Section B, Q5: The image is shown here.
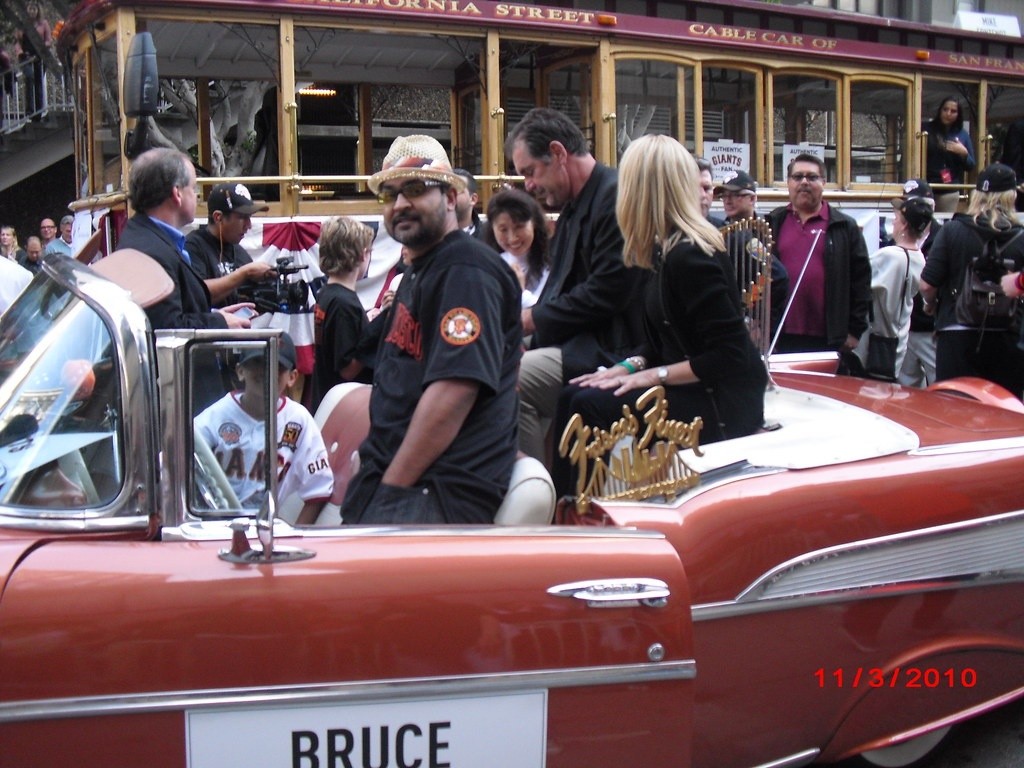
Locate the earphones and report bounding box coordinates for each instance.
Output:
[216,214,221,223]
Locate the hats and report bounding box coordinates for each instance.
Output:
[239,328,296,372]
[977,161,1018,192]
[207,179,269,215]
[713,170,757,191]
[367,134,467,193]
[899,178,933,198]
[891,196,933,231]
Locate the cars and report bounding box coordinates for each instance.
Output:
[0,252,1023,768]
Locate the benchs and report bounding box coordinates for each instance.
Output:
[276,381,556,526]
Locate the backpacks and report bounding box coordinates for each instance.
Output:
[945,216,1024,352]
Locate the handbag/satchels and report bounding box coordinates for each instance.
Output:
[866,329,899,381]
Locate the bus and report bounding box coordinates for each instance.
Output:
[50,1,1024,264]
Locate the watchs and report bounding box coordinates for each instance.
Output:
[655,364,669,385]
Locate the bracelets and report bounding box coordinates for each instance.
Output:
[615,360,635,373]
[625,357,645,370]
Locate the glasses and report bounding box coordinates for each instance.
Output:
[718,193,750,202]
[377,179,448,204]
[789,173,823,182]
[39,226,57,230]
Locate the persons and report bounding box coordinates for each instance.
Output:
[690,156,789,320]
[762,153,872,375]
[341,133,521,526]
[508,108,663,464]
[184,182,276,308]
[13,2,53,122]
[0,226,26,264]
[917,94,976,214]
[46,215,74,260]
[192,327,335,525]
[859,195,932,380]
[18,235,47,274]
[544,132,768,508]
[1000,268,1024,298]
[119,149,257,329]
[712,169,766,231]
[38,218,58,252]
[884,179,945,388]
[921,163,1024,400]
[313,166,559,386]
